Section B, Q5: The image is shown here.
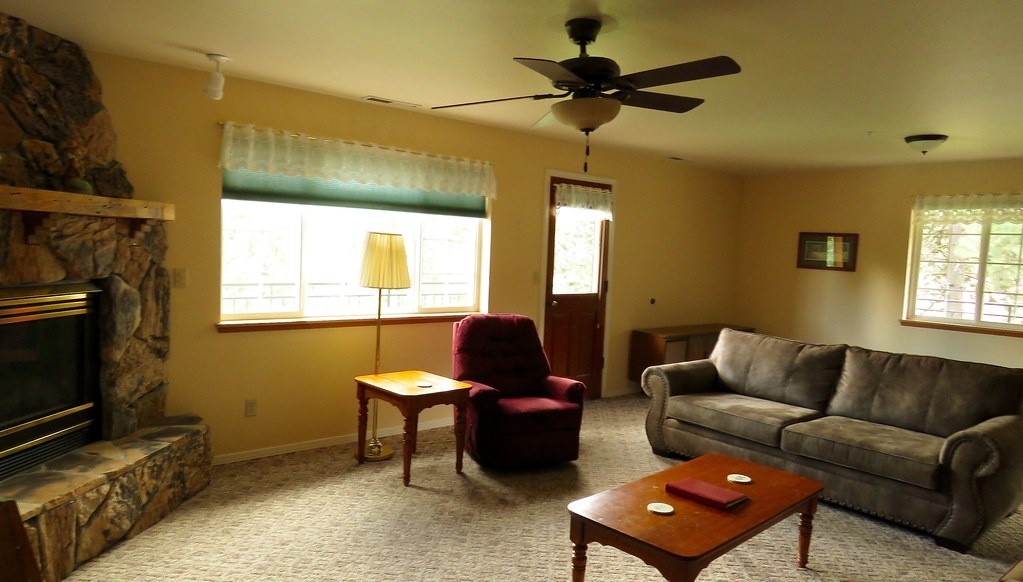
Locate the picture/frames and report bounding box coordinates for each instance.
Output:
[794,231,860,271]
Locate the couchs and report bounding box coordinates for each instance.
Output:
[451,310,586,477]
[640,309,1023,552]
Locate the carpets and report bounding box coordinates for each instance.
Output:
[61,380,1022,582]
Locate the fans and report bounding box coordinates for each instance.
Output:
[431,0,744,178]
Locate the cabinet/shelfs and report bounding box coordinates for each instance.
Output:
[627,321,755,389]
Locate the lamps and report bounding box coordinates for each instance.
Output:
[352,232,413,462]
[202,52,231,101]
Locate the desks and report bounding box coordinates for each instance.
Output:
[354,368,473,488]
[565,452,827,581]
